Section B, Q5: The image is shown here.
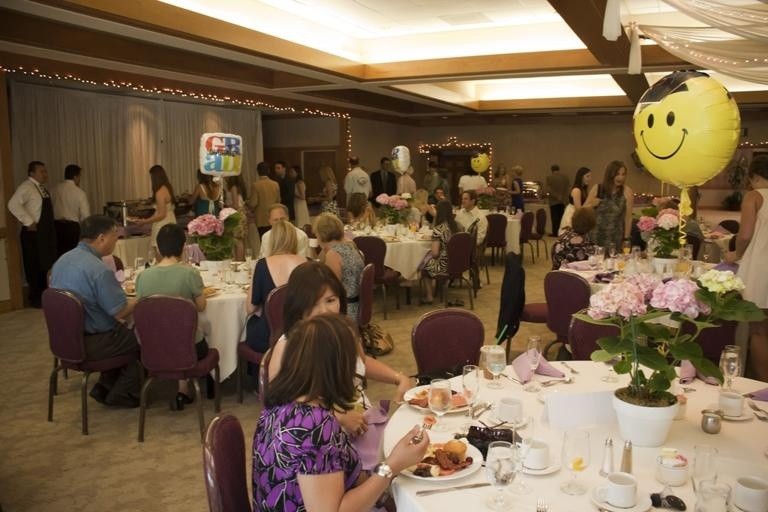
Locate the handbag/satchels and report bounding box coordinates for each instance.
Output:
[359,320,394,356]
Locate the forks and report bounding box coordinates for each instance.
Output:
[408,423,432,445]
[747,400,768,415]
[534,496,548,512]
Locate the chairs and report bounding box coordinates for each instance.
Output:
[544,270,590,360]
[411,307,485,387]
[202,410,253,512]
[299,209,549,320]
[568,316,621,360]
[42,243,375,441]
[495,252,549,365]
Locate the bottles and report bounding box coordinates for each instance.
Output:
[619,439,634,474]
[658,448,690,487]
[695,479,732,511]
[599,436,613,476]
[673,393,688,421]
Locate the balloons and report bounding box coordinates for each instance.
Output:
[632,70,741,246]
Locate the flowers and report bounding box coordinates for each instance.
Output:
[376,192,416,225]
[631,207,685,257]
[476,186,496,209]
[187,209,247,261]
[571,269,767,399]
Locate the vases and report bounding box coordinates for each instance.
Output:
[199,259,233,283]
[652,257,681,277]
[612,386,680,448]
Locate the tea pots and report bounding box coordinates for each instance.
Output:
[692,443,718,491]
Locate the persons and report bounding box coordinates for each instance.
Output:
[544,159,705,271]
[726,155,768,382]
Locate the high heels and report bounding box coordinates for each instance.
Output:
[175,393,193,410]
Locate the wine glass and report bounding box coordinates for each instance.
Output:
[485,440,518,510]
[487,345,507,389]
[120,248,254,294]
[603,351,622,384]
[558,429,593,495]
[588,241,705,279]
[719,342,743,392]
[460,364,480,430]
[429,379,452,433]
[523,336,543,392]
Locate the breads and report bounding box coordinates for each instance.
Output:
[443,440,467,462]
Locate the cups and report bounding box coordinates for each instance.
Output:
[498,398,522,424]
[479,346,500,381]
[719,390,744,418]
[701,407,723,434]
[733,477,768,512]
[598,471,638,509]
[521,437,549,469]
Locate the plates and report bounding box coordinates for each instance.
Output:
[400,435,484,482]
[590,484,652,511]
[518,450,560,474]
[402,384,477,415]
[720,408,754,422]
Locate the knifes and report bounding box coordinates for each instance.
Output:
[416,483,493,500]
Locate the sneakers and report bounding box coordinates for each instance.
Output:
[89,384,141,408]
[420,297,432,305]
